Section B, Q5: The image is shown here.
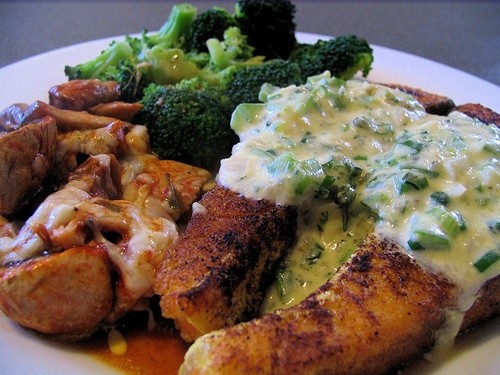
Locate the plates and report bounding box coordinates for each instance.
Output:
[0,31,500,375]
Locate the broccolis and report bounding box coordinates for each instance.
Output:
[62,0,374,171]
